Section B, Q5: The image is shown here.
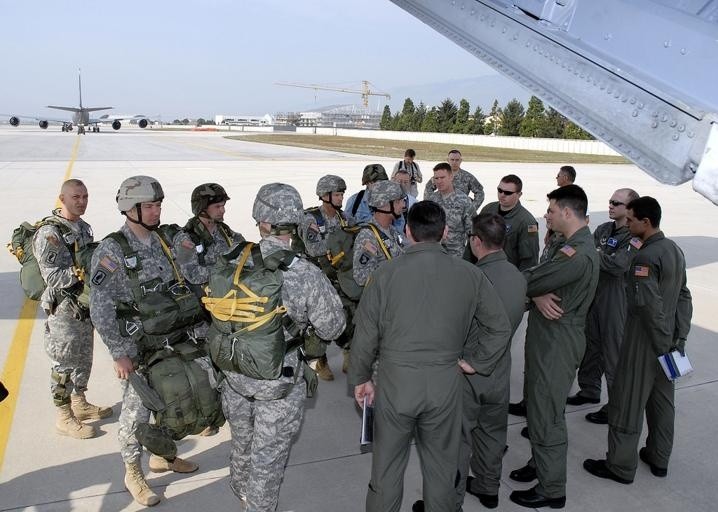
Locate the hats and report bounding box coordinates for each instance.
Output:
[315,175,348,198]
[367,181,407,207]
[191,182,232,212]
[361,163,389,185]
[250,182,308,224]
[117,175,165,212]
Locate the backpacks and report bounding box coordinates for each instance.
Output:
[327,221,391,300]
[9,216,75,299]
[78,230,139,311]
[205,243,307,381]
[161,221,199,244]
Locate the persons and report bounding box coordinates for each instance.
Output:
[349,200,526,512]
[90,175,347,512]
[31,179,113,440]
[295,149,540,454]
[508,164,692,510]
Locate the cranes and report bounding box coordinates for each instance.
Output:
[270,78,394,114]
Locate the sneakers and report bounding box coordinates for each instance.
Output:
[342,350,351,372]
[308,355,336,383]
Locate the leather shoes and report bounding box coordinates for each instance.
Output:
[566,392,602,407]
[640,446,667,477]
[510,485,565,510]
[509,466,536,482]
[583,456,635,486]
[466,473,500,509]
[508,399,528,417]
[585,409,611,426]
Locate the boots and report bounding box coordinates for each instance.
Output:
[54,404,97,440]
[123,460,161,509]
[147,452,201,475]
[71,393,113,420]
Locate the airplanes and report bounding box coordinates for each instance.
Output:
[0,68,164,133]
[381,1,718,214]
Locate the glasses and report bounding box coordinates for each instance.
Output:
[608,198,627,207]
[498,187,517,196]
